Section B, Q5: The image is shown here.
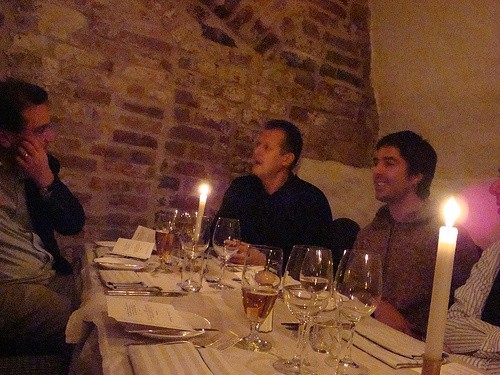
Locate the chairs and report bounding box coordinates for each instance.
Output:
[329,218,361,279]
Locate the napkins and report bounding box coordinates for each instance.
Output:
[97,269,162,293]
[338,320,450,370]
[127,343,237,375]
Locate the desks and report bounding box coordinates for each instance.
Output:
[65,244,491,375]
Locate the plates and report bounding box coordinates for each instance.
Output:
[220,272,281,295]
[277,285,344,312]
[121,310,212,339]
[93,258,149,271]
[94,241,117,247]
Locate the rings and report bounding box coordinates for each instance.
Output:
[23,152,28,157]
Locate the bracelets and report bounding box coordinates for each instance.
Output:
[40,180,57,195]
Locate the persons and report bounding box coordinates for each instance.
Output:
[0,76,84,342]
[444,169,500,373]
[349,129,482,339]
[210,119,333,275]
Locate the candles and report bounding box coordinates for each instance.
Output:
[194,182,211,241]
[420,195,461,375]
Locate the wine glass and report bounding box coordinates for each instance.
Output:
[236,245,283,352]
[209,217,241,290]
[272,244,383,375]
[152,207,177,274]
[174,211,212,288]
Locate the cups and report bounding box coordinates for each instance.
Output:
[206,244,222,282]
[182,251,205,292]
[225,241,250,272]
[310,316,343,354]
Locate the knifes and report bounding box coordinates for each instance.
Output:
[125,329,220,333]
[107,290,189,296]
[281,322,355,326]
[286,326,354,330]
